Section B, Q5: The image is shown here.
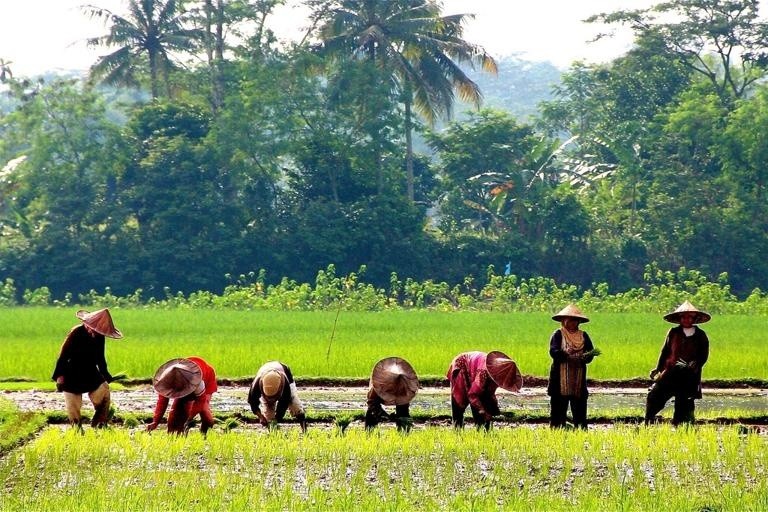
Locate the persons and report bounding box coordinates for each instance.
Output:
[547,304,594,432]
[52,307,124,430]
[645,300,711,427]
[447,350,523,431]
[248,361,306,433]
[146,356,217,434]
[365,356,420,433]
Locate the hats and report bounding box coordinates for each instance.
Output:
[76,308,123,339]
[372,357,419,406]
[263,372,281,396]
[153,358,202,400]
[552,304,589,323]
[485,351,522,392]
[664,300,711,324]
